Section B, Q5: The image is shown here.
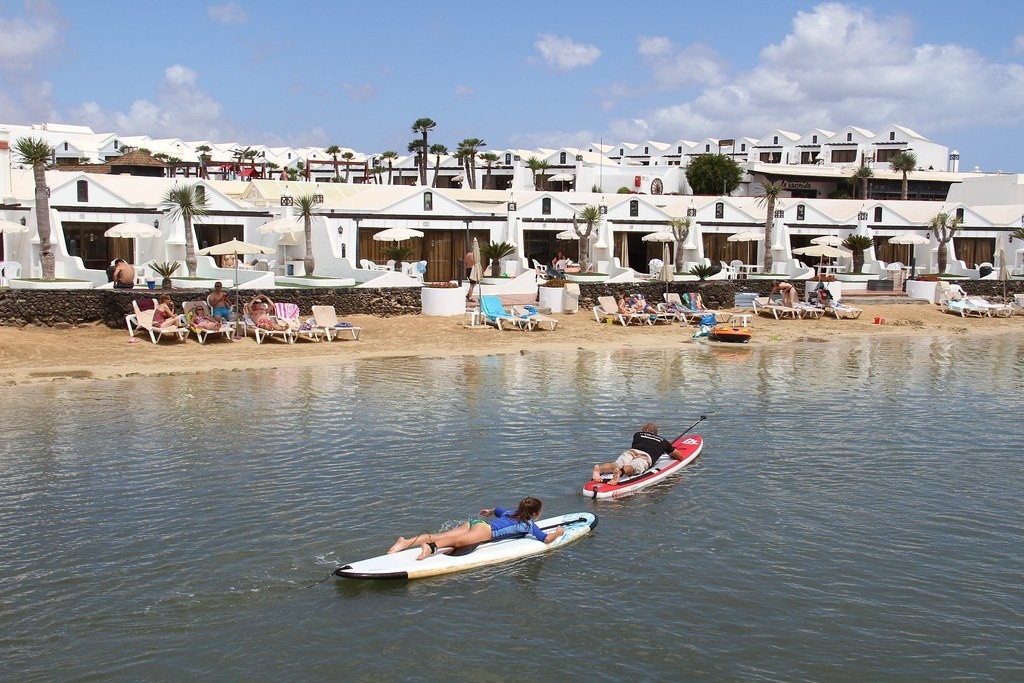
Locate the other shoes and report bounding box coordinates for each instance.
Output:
[466,294,476,302]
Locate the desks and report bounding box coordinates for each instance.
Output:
[733,315,754,328]
[464,312,486,328]
[223,320,247,337]
[813,265,845,277]
[806,303,822,317]
[740,265,763,273]
[902,266,925,279]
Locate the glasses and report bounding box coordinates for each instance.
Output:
[197,309,204,311]
[215,285,220,287]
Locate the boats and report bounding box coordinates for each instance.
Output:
[579,430,707,502]
[336,510,603,582]
[708,325,752,342]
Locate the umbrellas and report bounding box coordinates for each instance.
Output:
[791,234,852,309]
[641,230,683,265]
[547,170,575,191]
[0,218,29,234]
[195,237,276,338]
[888,233,930,269]
[659,243,674,313]
[372,227,424,250]
[727,229,765,273]
[556,227,597,263]
[257,218,314,276]
[993,233,1012,307]
[463,236,494,330]
[104,221,161,265]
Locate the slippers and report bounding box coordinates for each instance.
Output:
[128,339,139,343]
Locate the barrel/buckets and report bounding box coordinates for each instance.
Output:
[606,319,612,326]
[874,317,880,324]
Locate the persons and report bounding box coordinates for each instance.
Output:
[552,252,566,270]
[386,495,564,561]
[113,254,288,340]
[768,256,833,318]
[464,245,477,302]
[592,423,683,486]
[618,294,658,326]
[281,167,288,181]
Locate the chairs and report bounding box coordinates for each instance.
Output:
[478,296,558,332]
[360,258,427,282]
[125,298,361,345]
[111,258,155,285]
[0,262,22,287]
[944,291,1024,318]
[800,262,809,268]
[532,259,547,272]
[593,293,733,326]
[556,258,570,270]
[720,259,744,280]
[648,259,662,275]
[753,297,863,320]
